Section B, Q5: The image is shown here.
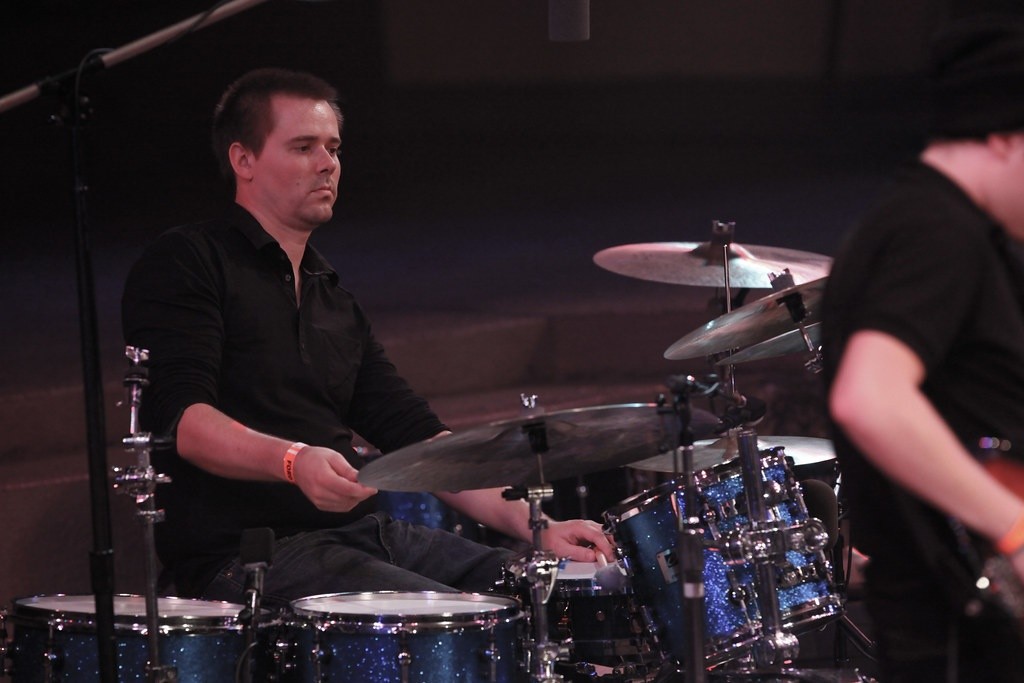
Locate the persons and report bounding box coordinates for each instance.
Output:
[816,79,1024,683]
[121,68,621,660]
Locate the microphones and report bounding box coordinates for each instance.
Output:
[241,527,275,606]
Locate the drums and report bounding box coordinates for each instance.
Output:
[11,452,842,682]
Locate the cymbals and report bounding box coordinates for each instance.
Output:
[357,244,835,496]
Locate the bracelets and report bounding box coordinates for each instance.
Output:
[997,517,1024,556]
[284,442,307,482]
